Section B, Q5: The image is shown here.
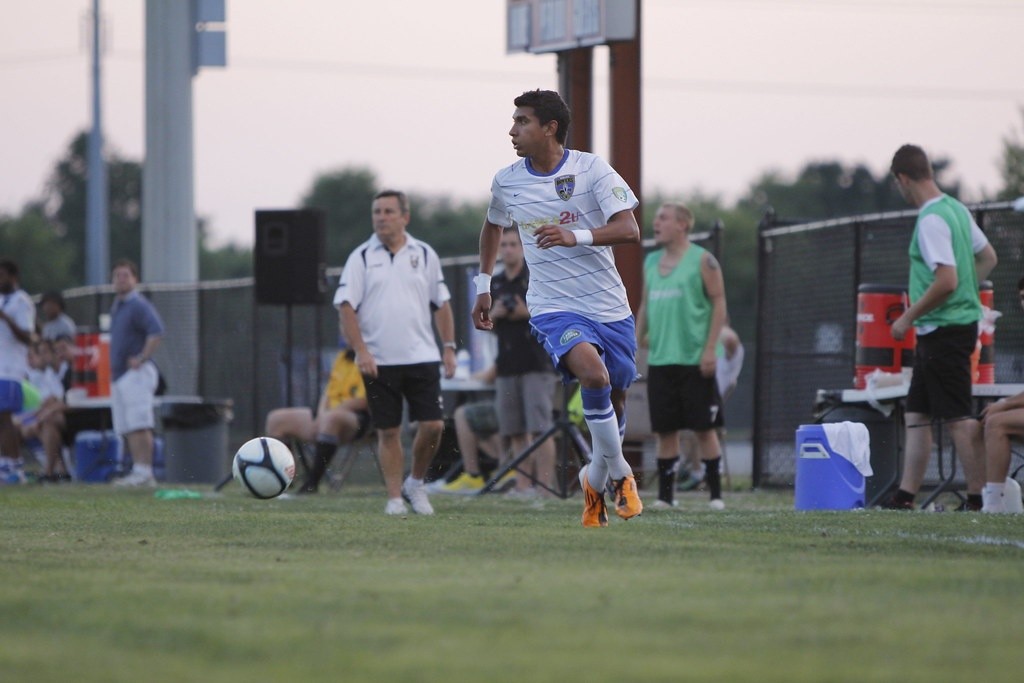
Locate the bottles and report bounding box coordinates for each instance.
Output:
[455,347,471,377]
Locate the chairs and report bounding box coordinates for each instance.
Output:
[287,426,387,492]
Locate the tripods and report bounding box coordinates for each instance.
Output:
[477,381,617,502]
[213,306,312,493]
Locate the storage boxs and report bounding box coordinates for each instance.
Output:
[69,432,163,480]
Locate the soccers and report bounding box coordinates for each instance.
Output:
[231,435,295,500]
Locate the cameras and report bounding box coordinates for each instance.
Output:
[499,294,518,312]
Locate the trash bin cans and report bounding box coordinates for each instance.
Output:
[795,423,866,512]
[153,395,233,484]
[63,397,123,484]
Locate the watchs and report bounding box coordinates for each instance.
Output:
[443,342,456,349]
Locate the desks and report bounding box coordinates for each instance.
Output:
[439,376,497,481]
[815,383,1024,510]
[67,394,234,409]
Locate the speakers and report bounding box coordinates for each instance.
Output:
[253,209,327,306]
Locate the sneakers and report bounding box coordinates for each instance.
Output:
[579,464,610,528]
[606,472,642,521]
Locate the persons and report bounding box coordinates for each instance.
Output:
[427,90,644,529]
[635,201,745,512]
[107,259,163,488]
[0,261,29,486]
[263,185,458,517]
[979,281,1024,513]
[876,145,998,510]
[8,290,113,485]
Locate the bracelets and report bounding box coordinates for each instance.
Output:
[571,229,593,246]
[472,273,492,295]
[137,353,145,362]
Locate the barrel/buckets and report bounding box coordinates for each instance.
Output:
[978,281,995,384]
[75,432,123,482]
[855,284,914,388]
[795,424,865,511]
[96,334,111,396]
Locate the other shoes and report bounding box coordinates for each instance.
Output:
[57,473,72,484]
[401,478,432,515]
[710,499,724,511]
[954,500,982,512]
[0,465,10,480]
[494,470,518,489]
[38,475,57,484]
[385,499,406,515]
[6,472,27,484]
[444,472,485,495]
[425,479,445,492]
[113,472,156,486]
[650,499,677,511]
[882,497,914,511]
